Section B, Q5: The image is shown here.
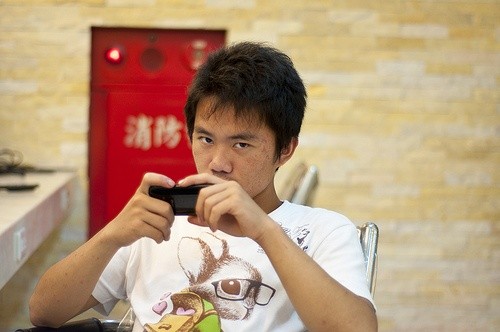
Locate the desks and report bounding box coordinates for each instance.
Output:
[0,169,77,291]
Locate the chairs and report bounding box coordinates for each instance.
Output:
[281,161,319,207]
[15,222,379,332]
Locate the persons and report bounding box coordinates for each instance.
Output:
[28,41,379,332]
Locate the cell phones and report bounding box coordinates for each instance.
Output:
[149,184,209,216]
